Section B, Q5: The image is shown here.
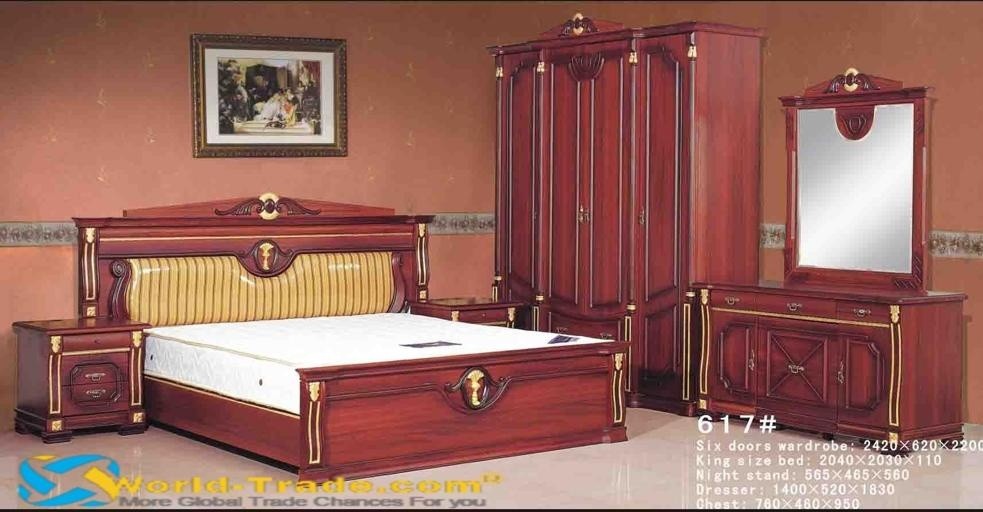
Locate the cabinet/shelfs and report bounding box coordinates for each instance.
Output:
[485,11,969,459]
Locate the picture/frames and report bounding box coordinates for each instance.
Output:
[190,32,349,158]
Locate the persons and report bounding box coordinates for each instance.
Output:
[251,79,314,128]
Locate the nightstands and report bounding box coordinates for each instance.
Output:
[11,318,152,444]
[407,297,525,328]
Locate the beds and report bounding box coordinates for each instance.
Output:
[70,192,633,488]
